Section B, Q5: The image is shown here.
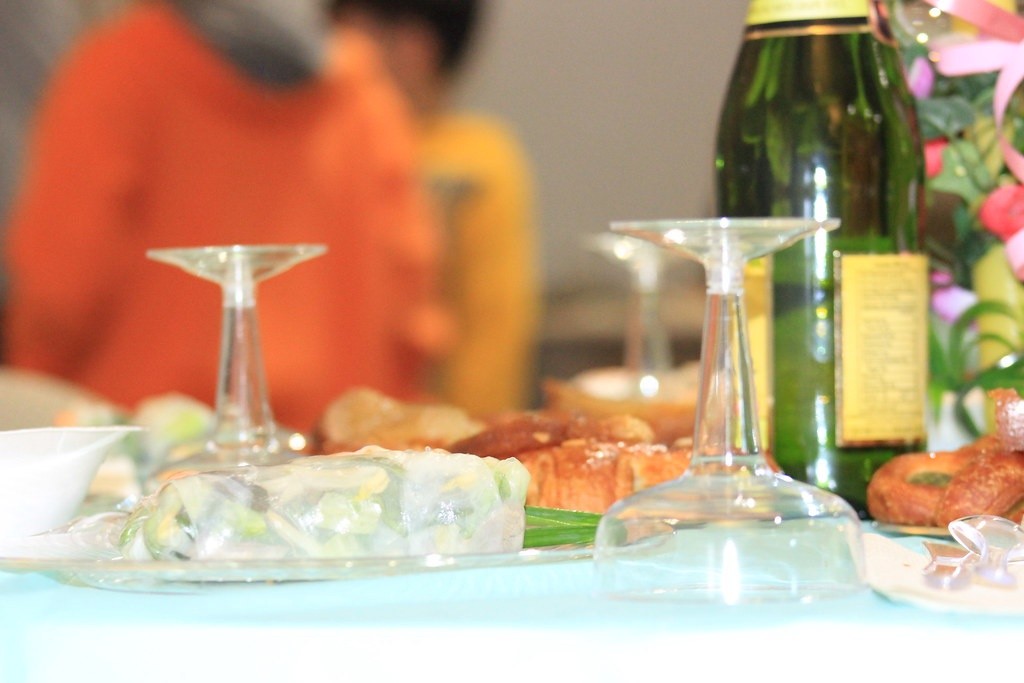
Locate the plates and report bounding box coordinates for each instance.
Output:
[0,516,675,584]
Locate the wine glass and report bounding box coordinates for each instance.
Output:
[141,245,312,497]
[592,216,866,599]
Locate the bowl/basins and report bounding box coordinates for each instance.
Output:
[0,425,129,537]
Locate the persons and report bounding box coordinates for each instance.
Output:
[0,0,436,450]
[321,1,539,423]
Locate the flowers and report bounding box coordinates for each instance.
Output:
[874,0,1023,440]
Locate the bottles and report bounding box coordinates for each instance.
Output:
[705,0,931,522]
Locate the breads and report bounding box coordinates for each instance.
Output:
[866,389,1023,533]
[318,387,785,518]
